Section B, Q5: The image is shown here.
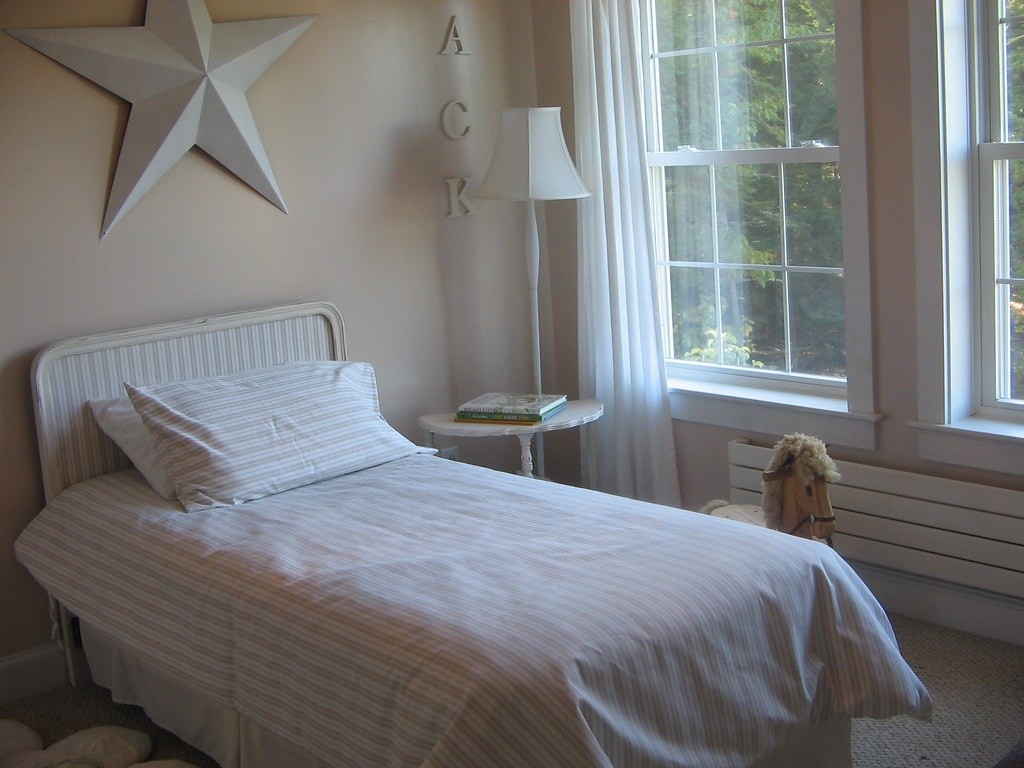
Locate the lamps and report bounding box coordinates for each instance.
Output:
[468,107,594,480]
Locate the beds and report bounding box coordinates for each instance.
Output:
[12,299,934,768]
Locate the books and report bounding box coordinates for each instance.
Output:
[454,418,540,427]
[459,393,567,414]
[456,402,570,421]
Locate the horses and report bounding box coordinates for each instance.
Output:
[696,432,843,549]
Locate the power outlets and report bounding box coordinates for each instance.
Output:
[441,446,460,462]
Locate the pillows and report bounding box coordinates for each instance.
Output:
[124,361,438,513]
[85,397,179,502]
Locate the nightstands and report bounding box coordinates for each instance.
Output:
[419,400,604,491]
